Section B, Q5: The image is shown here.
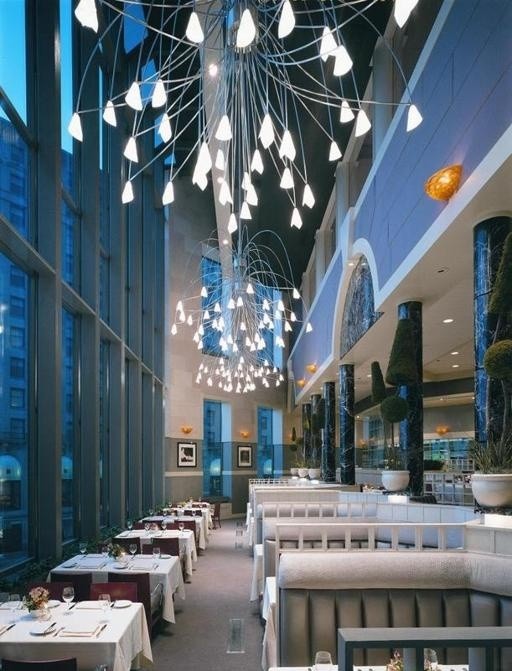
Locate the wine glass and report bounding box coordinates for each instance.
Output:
[130,545,137,561]
[98,594,111,624]
[128,497,203,538]
[62,588,75,617]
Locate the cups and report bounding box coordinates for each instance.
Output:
[79,544,88,554]
[152,548,161,559]
[315,652,333,671]
[423,648,438,671]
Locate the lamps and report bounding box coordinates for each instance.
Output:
[423,162,463,202]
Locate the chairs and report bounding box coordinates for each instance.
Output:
[0,499,222,671]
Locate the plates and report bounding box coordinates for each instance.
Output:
[29,624,56,635]
[111,600,132,609]
[112,562,130,570]
[47,600,61,609]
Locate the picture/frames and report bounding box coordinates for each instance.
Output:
[176,441,197,469]
[236,445,252,468]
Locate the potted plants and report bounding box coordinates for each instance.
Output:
[465,428,512,509]
[380,437,414,492]
[289,455,321,480]
